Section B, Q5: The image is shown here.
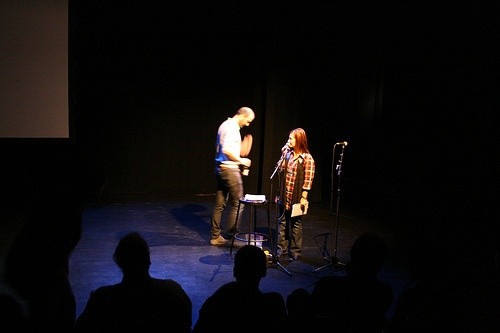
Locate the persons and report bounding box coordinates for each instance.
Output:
[0,203,500,333]
[270,127,315,262]
[209,107,255,245]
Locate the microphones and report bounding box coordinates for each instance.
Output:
[284,143,290,158]
[336,141,347,146]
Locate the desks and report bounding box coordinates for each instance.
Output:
[229,197,273,259]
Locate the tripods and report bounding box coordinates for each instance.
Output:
[312,145,348,273]
[266,154,293,277]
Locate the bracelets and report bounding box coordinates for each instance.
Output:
[302,196,307,200]
